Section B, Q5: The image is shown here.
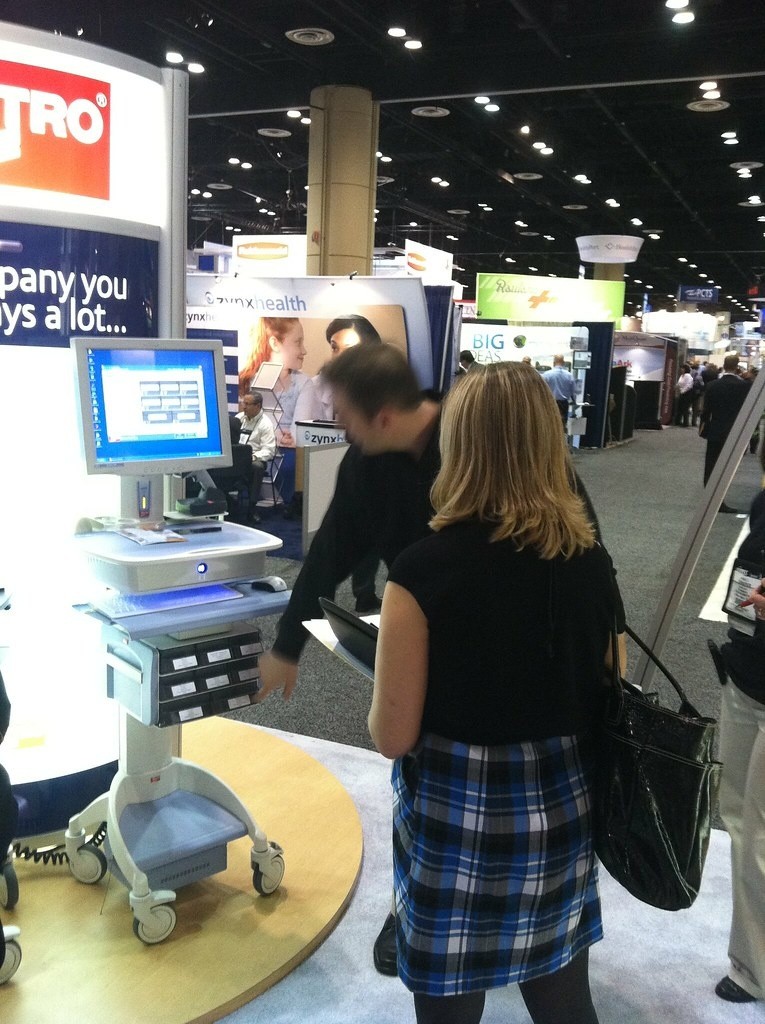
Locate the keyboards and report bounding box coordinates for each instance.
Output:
[86,583,244,619]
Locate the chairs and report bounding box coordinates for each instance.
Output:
[262,454,285,509]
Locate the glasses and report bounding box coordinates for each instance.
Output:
[243,402,257,405]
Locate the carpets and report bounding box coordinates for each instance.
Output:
[209,712,765,1024]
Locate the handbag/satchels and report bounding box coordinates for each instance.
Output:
[699,420,711,438]
[593,540,725,912]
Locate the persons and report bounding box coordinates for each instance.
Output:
[239,317,308,448]
[522,356,531,364]
[290,314,381,445]
[235,391,276,523]
[688,359,759,426]
[460,350,484,370]
[700,355,749,512]
[367,362,626,1024]
[253,347,445,705]
[676,364,694,427]
[542,354,576,423]
[714,488,765,1002]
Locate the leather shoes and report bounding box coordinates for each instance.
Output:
[373,912,398,976]
[248,511,261,523]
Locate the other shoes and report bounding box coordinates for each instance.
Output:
[692,422,698,426]
[716,976,755,1002]
[682,422,690,427]
[718,503,737,513]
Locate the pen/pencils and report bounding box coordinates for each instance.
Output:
[172,526,222,535]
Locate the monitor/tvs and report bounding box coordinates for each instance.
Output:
[71,335,233,533]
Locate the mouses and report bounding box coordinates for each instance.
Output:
[252,576,287,593]
